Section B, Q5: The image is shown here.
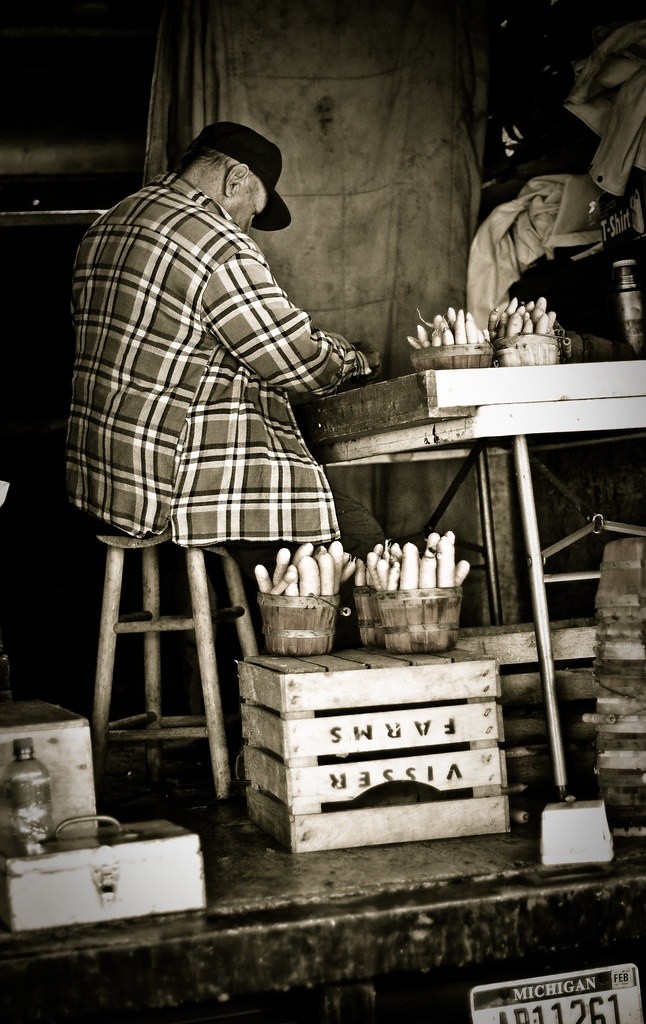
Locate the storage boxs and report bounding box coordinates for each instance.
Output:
[0,699,100,833]
[241,648,511,856]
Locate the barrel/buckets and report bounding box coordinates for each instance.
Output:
[409,344,494,372]
[582,537,646,838]
[257,591,351,656]
[353,586,464,656]
[492,329,571,368]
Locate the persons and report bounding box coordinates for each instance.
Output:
[67,120,384,649]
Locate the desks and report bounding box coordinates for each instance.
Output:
[319,395,646,800]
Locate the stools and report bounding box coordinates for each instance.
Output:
[94,523,259,797]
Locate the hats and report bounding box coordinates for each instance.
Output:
[188,122,291,231]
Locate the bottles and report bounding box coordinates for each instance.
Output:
[0,641,12,704]
[4,736,53,855]
